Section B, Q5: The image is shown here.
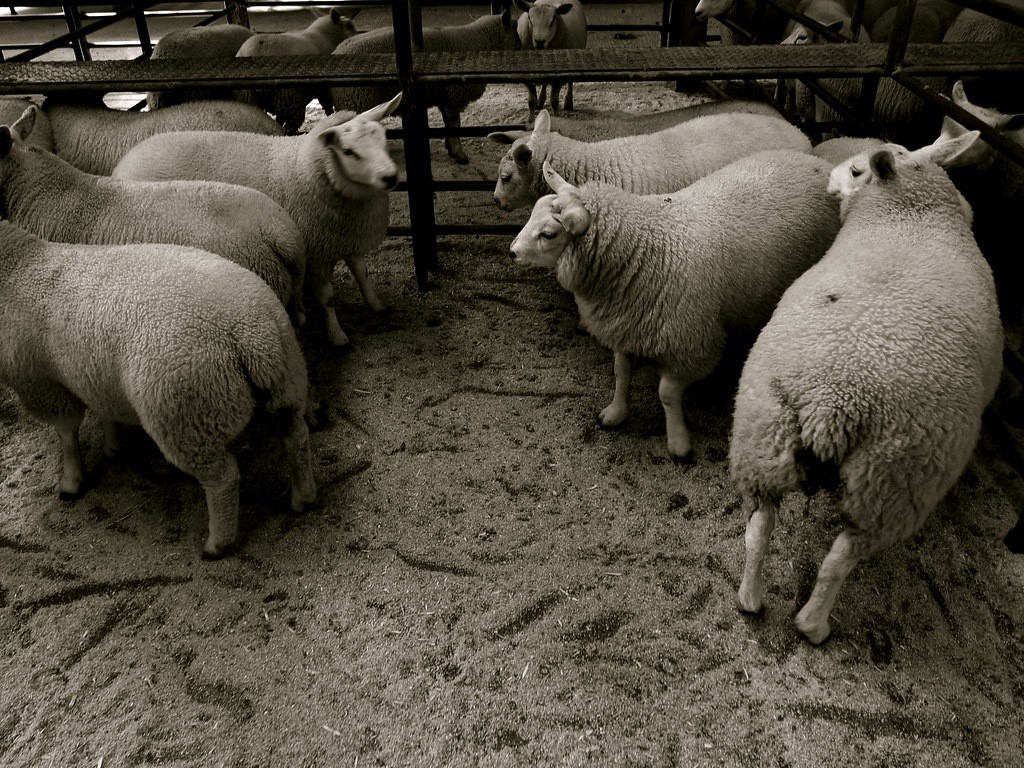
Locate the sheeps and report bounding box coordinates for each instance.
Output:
[695,1,1024,141]
[515,1,587,117]
[0,218,323,562]
[38,89,287,177]
[486,99,787,146]
[726,131,1006,646]
[509,149,842,464]
[1,98,58,155]
[145,24,258,112]
[0,105,331,434]
[235,7,359,135]
[112,89,404,351]
[934,80,1023,201]
[492,108,816,211]
[816,136,969,234]
[325,8,525,165]
